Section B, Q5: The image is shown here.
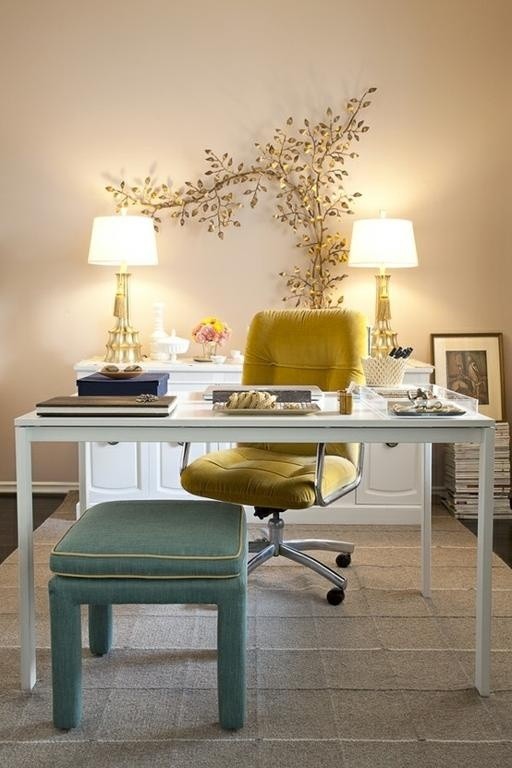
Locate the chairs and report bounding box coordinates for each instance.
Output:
[181,309,372,607]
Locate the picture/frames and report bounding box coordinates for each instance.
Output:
[430,331,506,425]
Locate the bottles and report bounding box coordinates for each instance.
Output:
[341,393,352,414]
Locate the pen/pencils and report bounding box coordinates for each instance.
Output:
[389,347,414,358]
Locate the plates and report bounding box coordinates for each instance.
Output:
[97,372,144,379]
[212,402,321,414]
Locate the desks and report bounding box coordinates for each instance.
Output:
[13,390,499,697]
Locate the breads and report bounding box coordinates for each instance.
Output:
[226,389,279,412]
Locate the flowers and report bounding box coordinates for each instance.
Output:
[193,316,231,346]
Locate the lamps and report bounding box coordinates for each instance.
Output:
[85,208,158,364]
[351,209,420,357]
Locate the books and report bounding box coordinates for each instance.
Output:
[440,421,512,521]
[35,395,178,417]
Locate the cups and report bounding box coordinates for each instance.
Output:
[230,350,241,357]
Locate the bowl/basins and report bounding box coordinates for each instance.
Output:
[211,356,227,364]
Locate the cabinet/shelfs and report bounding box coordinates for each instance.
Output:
[73,358,435,527]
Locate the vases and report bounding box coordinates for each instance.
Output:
[202,342,217,359]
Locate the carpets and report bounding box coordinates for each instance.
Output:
[0,493,511,768]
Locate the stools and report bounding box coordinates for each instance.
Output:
[47,497,256,732]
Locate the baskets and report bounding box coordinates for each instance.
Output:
[359,354,412,388]
